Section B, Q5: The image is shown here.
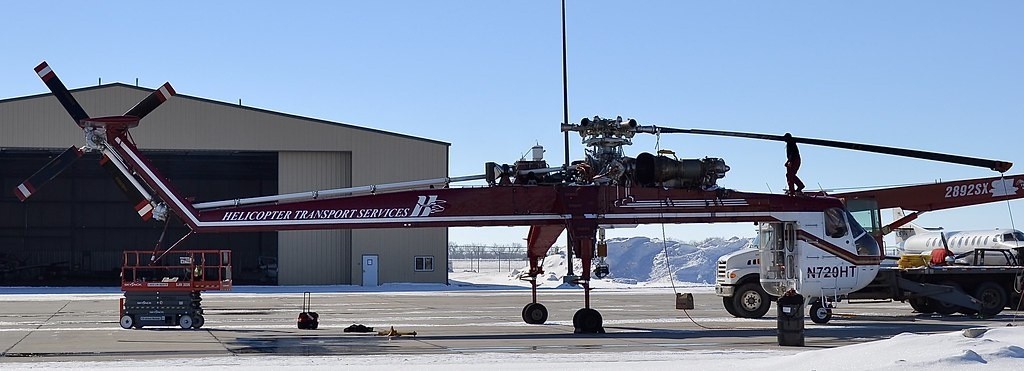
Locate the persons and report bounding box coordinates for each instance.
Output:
[593,151,624,185]
[193,253,205,280]
[783,134,804,193]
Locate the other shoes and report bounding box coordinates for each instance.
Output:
[796,183,805,191]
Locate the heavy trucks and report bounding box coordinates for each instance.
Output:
[715,205,1024,325]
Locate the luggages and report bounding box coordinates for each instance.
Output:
[297,292,319,329]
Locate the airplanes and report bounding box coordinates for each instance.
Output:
[13,60,1024,349]
